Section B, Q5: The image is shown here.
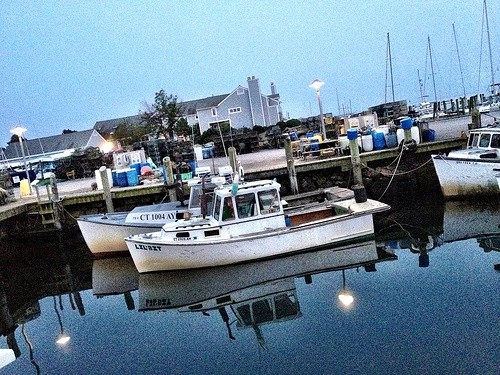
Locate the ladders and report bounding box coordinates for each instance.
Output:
[36,185,55,228]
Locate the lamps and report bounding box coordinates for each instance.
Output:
[10,125,27,137]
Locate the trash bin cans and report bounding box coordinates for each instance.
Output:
[111,162,150,187]
[201,148,212,159]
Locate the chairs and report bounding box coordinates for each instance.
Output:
[247,199,253,218]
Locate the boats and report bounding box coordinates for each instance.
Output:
[74,176,356,260]
[375,0,500,122]
[430,126,500,201]
[123,178,392,276]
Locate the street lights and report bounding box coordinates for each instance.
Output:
[308,79,330,141]
[10,125,33,196]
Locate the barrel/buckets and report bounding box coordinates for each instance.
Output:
[362,134,373,152]
[111,169,127,186]
[397,126,420,145]
[338,134,349,154]
[20,179,30,195]
[187,160,195,175]
[127,169,139,185]
[130,163,151,175]
[353,186,368,203]
[357,136,362,152]
[373,132,385,149]
